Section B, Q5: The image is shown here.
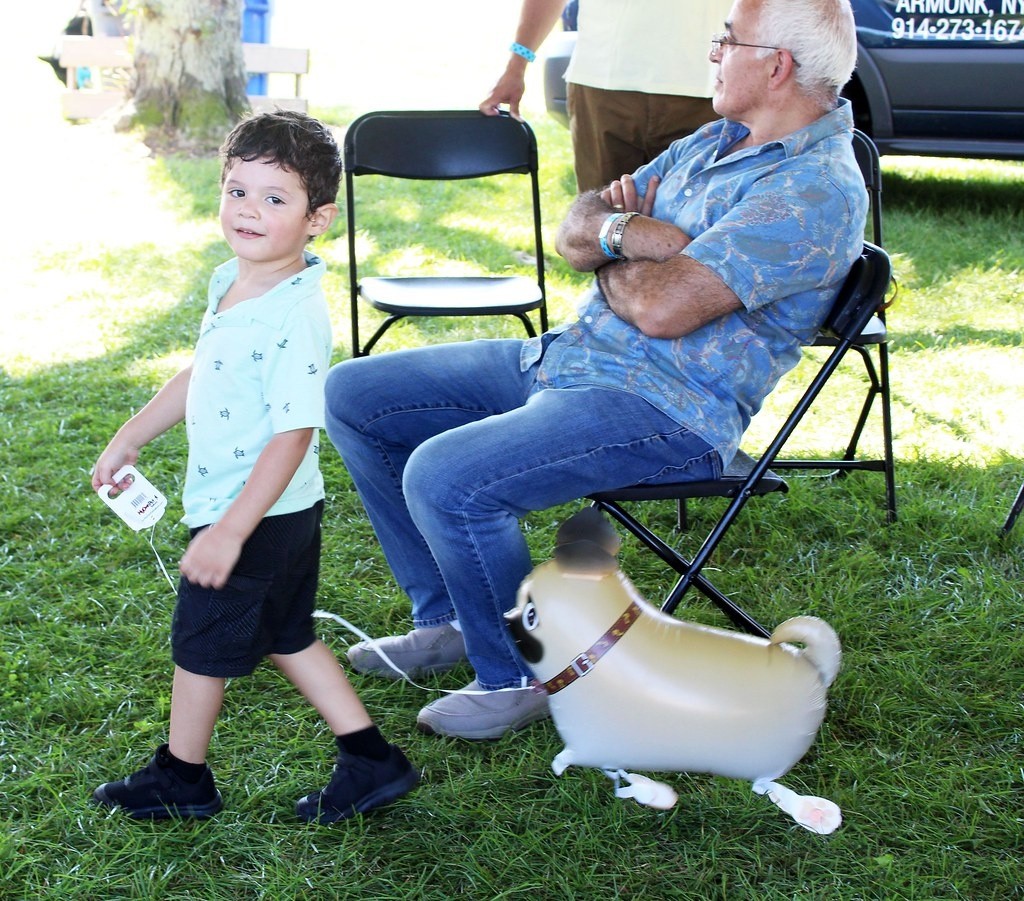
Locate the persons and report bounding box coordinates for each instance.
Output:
[479,1,731,197]
[345,0,869,742]
[90,110,417,824]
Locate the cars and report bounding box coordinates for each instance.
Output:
[541,0,1024,159]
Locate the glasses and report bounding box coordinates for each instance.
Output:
[711,33,801,69]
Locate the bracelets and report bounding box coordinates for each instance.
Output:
[598,213,623,261]
[510,42,536,62]
[612,211,639,258]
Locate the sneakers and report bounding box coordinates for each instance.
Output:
[348,622,473,678]
[297,743,416,823]
[93,743,222,819]
[417,679,552,739]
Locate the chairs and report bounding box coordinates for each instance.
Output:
[585,244,893,641]
[674,126,899,537]
[344,108,551,366]
